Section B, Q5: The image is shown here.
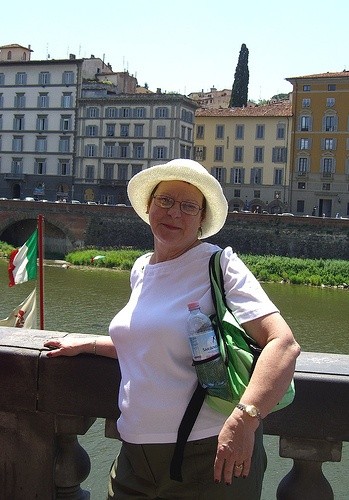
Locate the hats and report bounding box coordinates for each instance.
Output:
[127,159,229,240]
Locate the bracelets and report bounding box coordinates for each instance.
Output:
[92,338,97,356]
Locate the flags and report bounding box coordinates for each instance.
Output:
[8,228,38,286]
[0,287,36,329]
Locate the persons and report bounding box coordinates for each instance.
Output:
[42,158,300,500]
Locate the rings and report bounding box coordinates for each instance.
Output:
[235,464,243,471]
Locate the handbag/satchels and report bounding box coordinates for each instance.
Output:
[169,249,296,482]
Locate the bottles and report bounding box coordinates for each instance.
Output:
[185,302,228,388]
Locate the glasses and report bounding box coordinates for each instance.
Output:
[151,195,204,217]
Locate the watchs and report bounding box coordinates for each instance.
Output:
[236,403,262,424]
